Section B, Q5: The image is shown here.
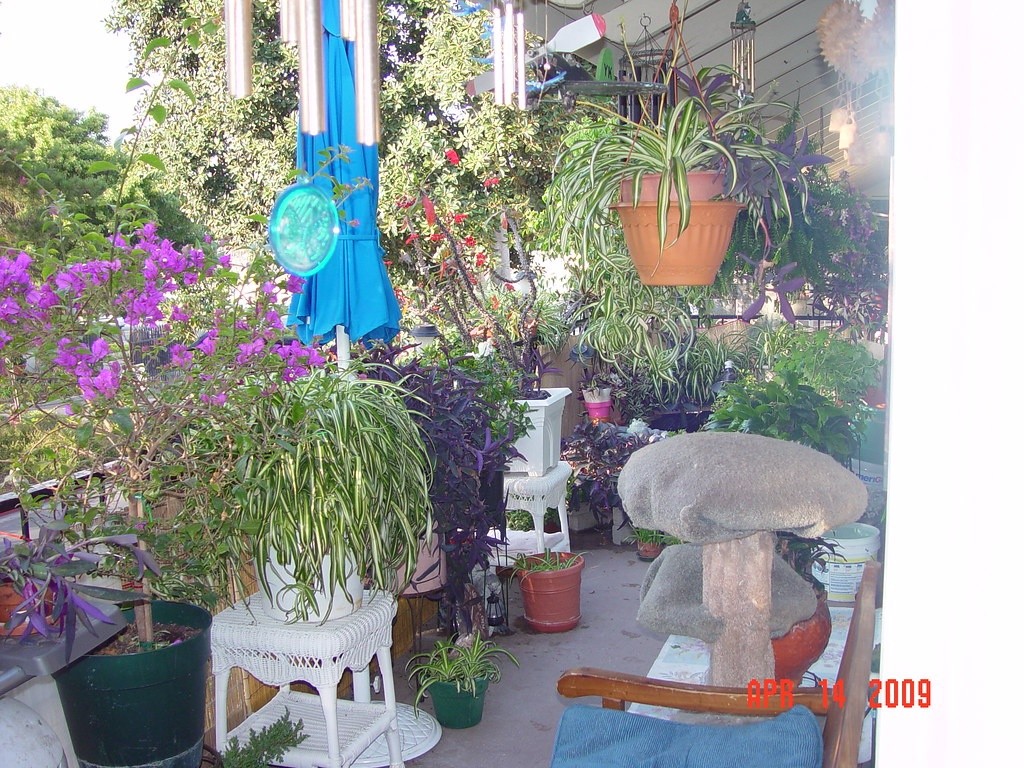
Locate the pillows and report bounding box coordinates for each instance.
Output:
[549,703,825,768]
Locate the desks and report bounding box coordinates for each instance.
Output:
[210,588,405,768]
[485,461,573,577]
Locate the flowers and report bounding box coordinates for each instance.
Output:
[0,217,325,644]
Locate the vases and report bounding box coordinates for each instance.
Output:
[609,203,746,287]
[585,401,612,423]
[53,599,214,768]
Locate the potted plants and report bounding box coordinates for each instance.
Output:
[573,423,680,559]
[0,531,162,666]
[407,627,522,729]
[579,376,612,403]
[496,347,572,477]
[218,341,527,628]
[552,63,836,405]
[701,377,856,690]
[499,547,587,632]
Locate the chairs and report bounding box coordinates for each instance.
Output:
[558,562,876,768]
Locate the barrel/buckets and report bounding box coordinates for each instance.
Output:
[811,522,881,601]
